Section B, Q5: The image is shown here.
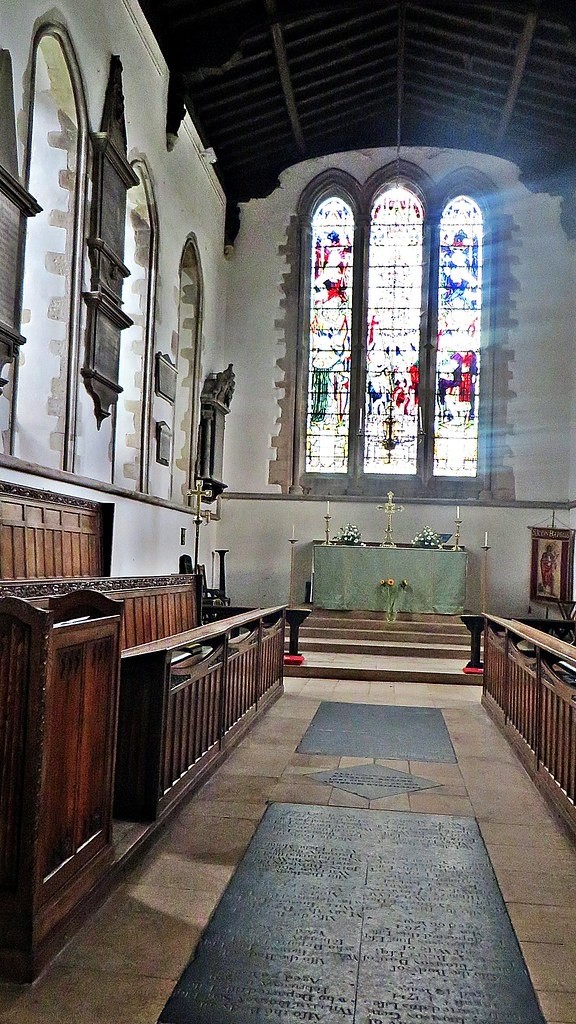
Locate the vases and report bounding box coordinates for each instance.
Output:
[386,599,397,622]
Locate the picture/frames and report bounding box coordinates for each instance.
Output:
[526,526,576,607]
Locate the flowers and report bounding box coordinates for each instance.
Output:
[333,522,363,543]
[410,525,443,549]
[381,578,409,620]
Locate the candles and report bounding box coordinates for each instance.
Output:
[457,505,460,519]
[485,530,488,546]
[327,500,330,514]
[292,525,295,539]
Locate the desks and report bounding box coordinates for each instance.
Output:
[312,544,468,616]
